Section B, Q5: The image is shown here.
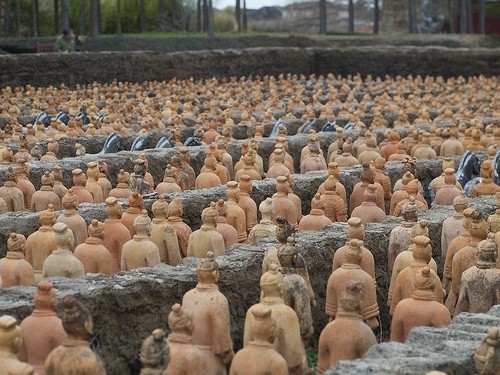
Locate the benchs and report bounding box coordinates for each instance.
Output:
[36,41,54,53]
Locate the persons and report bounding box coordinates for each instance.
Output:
[54,29,85,53]
[0,71,500,375]
